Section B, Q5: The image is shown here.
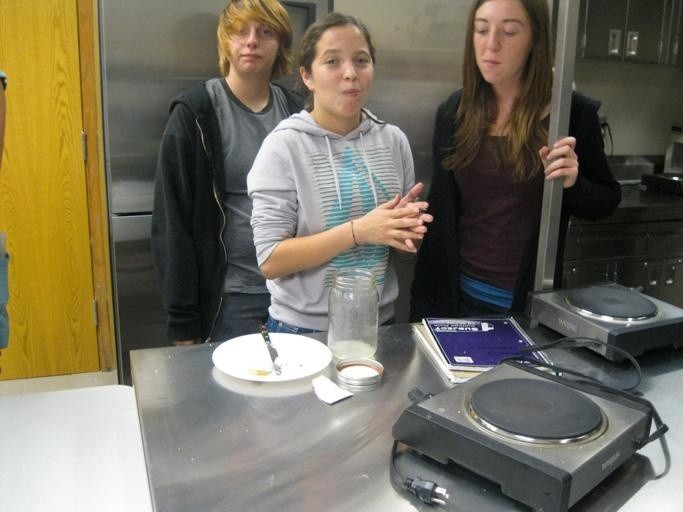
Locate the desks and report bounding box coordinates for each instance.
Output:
[127,300,683,512]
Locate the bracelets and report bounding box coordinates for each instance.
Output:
[349,219,360,248]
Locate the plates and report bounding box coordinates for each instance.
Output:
[212,332,333,382]
[212,365,331,399]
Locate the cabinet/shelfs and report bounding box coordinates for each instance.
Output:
[557,207,683,307]
[553,1,670,65]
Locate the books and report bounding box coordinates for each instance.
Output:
[413,316,564,386]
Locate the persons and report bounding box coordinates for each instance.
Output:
[246,10,435,336]
[148,0,294,345]
[408,0,623,321]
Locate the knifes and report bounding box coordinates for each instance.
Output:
[259,324,281,375]
